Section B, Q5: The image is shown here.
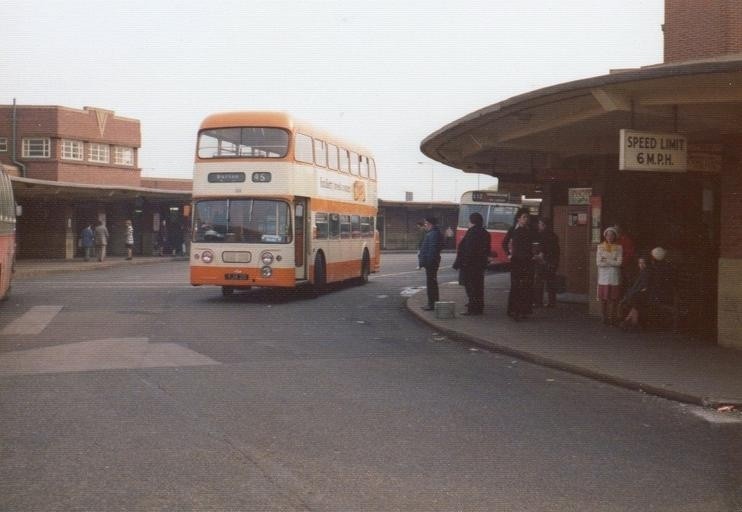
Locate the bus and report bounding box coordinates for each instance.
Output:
[189,112,380,296]
[1,163,23,300]
[455,190,543,273]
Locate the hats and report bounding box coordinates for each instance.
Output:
[603,226,619,240]
[650,247,667,261]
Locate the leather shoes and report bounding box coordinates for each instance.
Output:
[459,303,473,314]
[420,304,435,310]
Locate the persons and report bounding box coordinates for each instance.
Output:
[502,208,536,319]
[534,217,560,309]
[595,221,674,333]
[159,219,167,256]
[94,219,109,262]
[452,212,491,315]
[123,219,134,260]
[416,221,425,270]
[418,217,441,310]
[79,224,94,261]
[444,226,454,244]
